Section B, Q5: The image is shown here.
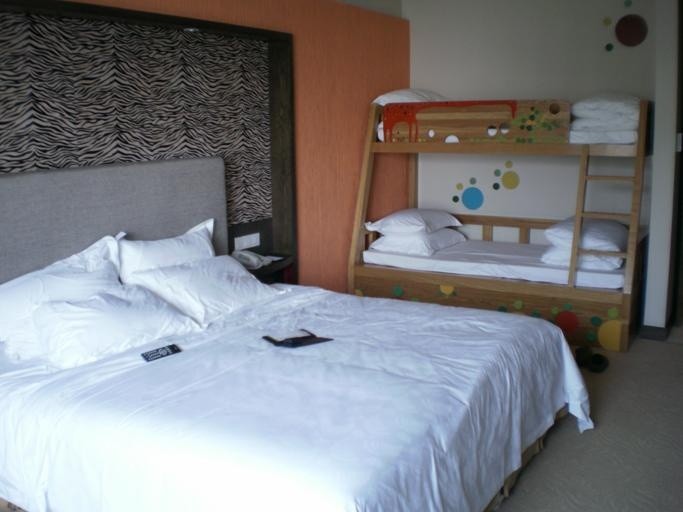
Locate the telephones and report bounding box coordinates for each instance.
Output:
[232,250,271,270]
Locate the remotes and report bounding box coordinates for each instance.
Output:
[140,343,182,363]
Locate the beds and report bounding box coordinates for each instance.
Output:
[345,91,650,356]
[0,155,596,512]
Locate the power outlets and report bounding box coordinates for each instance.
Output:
[231,231,260,248]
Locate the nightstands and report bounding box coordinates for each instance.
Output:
[229,252,293,286]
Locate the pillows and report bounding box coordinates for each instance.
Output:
[0,237,123,339]
[19,283,200,368]
[364,207,461,234]
[368,232,464,255]
[372,87,449,117]
[108,218,226,280]
[136,254,288,324]
